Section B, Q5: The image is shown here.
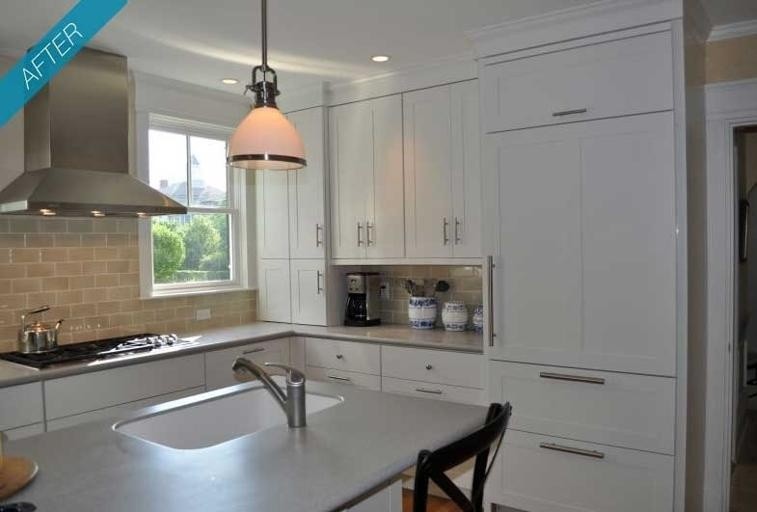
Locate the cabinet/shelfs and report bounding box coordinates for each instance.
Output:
[402,77,488,266]
[44,351,206,433]
[0,378,47,444]
[470,0,715,512]
[253,106,362,326]
[381,341,485,504]
[304,336,381,394]
[327,91,402,265]
[205,336,293,392]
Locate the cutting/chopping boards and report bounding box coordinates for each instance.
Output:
[0,451,40,499]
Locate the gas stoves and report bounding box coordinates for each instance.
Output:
[0,333,200,370]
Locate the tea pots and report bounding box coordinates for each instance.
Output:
[18,304,63,353]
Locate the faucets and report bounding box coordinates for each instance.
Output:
[233,357,307,427]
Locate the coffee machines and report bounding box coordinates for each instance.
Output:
[344,272,382,326]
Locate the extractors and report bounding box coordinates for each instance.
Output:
[0,41,188,221]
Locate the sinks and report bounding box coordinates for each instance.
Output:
[112,383,344,451]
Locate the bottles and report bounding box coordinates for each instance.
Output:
[408,295,483,332]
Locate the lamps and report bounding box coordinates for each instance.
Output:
[227,0,307,172]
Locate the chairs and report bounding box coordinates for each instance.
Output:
[410,400,511,512]
